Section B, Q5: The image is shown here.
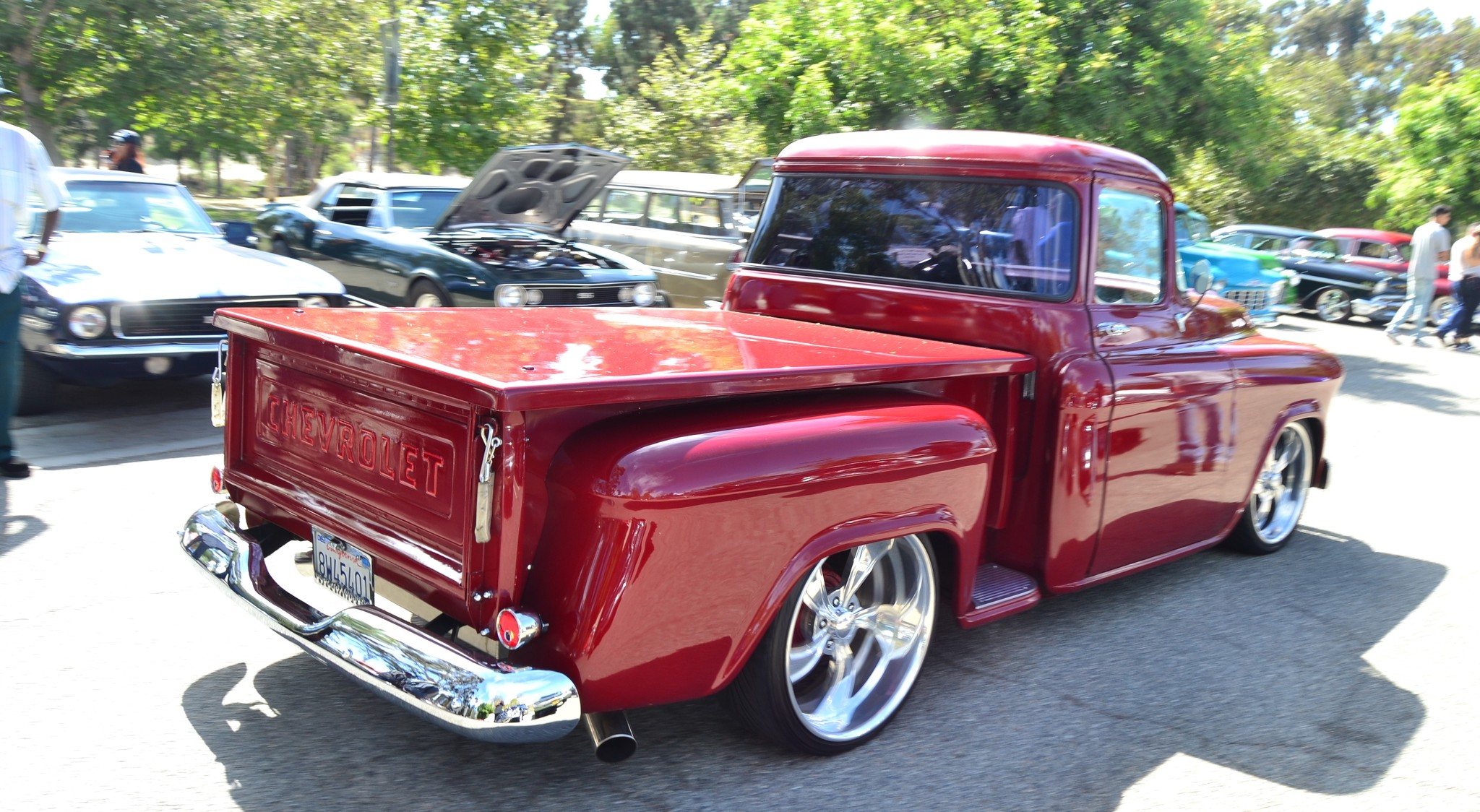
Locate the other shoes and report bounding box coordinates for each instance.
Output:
[1435,327,1445,344]
[1384,331,1401,345]
[1410,339,1432,349]
[1453,342,1479,353]
[0,455,30,478]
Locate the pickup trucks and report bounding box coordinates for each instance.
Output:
[179,128,1346,759]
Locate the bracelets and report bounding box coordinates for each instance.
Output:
[35,244,49,253]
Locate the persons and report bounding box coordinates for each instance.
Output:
[1385,204,1449,347]
[1435,223,1480,353]
[107,130,147,174]
[0,120,68,482]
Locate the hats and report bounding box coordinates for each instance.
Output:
[1434,204,1452,217]
[0,76,20,98]
[108,129,141,145]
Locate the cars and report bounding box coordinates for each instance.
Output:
[564,156,776,310]
[1207,222,1480,336]
[253,141,668,308]
[1097,188,1290,326]
[14,165,348,417]
